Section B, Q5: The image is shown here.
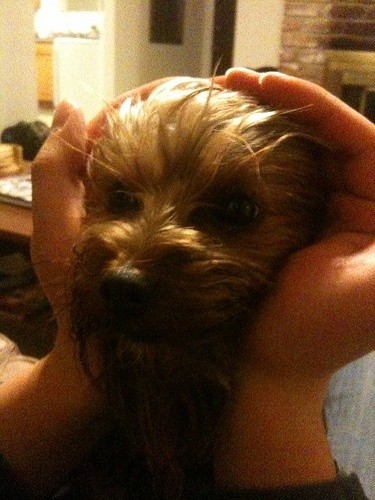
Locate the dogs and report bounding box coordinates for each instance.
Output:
[48,56,336,498]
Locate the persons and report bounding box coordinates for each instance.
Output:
[0,64,375,500]
